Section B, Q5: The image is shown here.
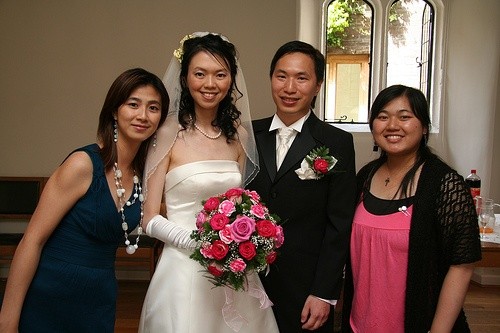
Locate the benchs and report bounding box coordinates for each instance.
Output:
[0,177,161,273]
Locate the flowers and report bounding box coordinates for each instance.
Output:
[304,146,335,180]
[191,187,285,332]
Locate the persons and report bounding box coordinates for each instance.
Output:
[349,85,481,333]
[138,31,279,333]
[244,41,356,333]
[0,68,170,333]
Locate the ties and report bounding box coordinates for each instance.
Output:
[274,127,296,173]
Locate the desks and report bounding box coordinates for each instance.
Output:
[464,211,500,332]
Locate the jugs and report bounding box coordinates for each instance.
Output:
[473,195,500,233]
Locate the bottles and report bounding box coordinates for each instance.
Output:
[465,169,480,219]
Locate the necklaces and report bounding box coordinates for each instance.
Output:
[381,163,412,187]
[110,159,143,255]
[189,114,223,140]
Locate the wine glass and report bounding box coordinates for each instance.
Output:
[480,210,491,239]
